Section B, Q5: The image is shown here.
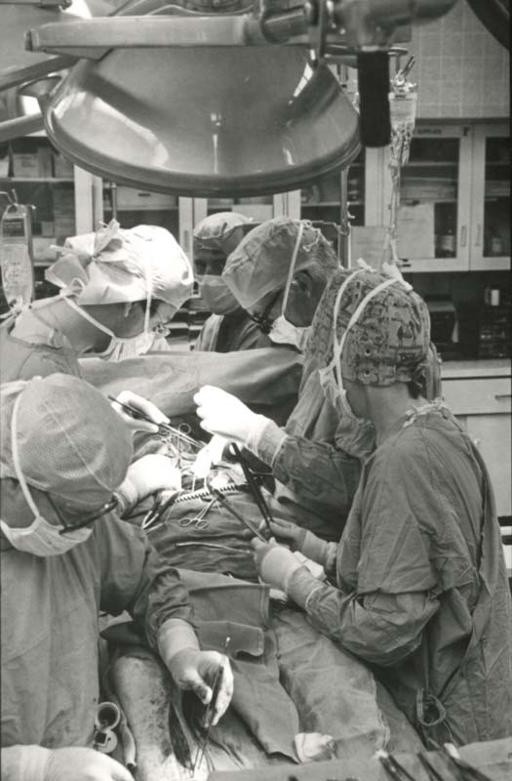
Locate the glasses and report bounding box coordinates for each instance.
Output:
[154,307,170,336]
[43,488,119,535]
[252,288,281,334]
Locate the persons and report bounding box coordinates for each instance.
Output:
[191,212,261,353]
[252,265,512,748]
[0,373,235,781]
[192,216,443,541]
[0,223,191,518]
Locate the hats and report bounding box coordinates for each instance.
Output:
[125,224,195,308]
[313,264,433,387]
[197,212,333,308]
[0,373,135,510]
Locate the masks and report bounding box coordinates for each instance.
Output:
[84,302,160,364]
[267,315,312,353]
[317,360,363,423]
[0,477,94,557]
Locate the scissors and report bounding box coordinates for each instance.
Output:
[158,423,192,468]
[179,498,217,531]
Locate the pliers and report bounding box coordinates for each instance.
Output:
[141,493,177,529]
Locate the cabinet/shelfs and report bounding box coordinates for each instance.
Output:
[0,129,91,265]
[384,123,512,273]
[436,377,511,521]
[286,122,380,268]
[89,161,285,280]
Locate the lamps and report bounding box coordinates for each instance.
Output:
[24,0,461,198]
